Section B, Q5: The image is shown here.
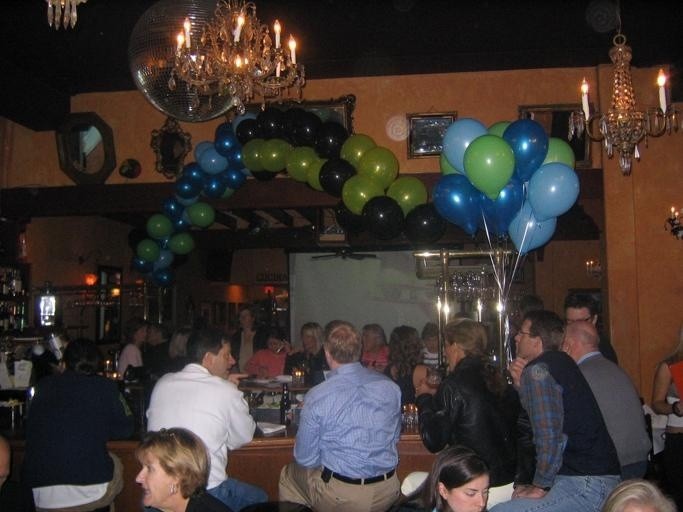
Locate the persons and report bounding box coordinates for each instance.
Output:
[1,288,683,511]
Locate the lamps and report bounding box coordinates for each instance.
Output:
[568,0,677,174]
[168,0,306,114]
[46,0,86,29]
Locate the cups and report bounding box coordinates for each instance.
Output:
[427,372,441,388]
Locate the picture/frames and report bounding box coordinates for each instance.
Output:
[224,96,356,134]
[148,118,192,178]
[518,104,592,167]
[407,112,457,159]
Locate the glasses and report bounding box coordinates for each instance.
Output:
[565,317,592,323]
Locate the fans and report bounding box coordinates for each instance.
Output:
[312,250,377,261]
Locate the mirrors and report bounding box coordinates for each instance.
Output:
[96,264,121,344]
[55,109,118,184]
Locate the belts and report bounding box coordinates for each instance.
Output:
[331,465,398,486]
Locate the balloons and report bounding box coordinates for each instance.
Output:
[132,103,580,286]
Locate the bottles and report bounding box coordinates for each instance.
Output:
[0,302,26,331]
[0,269,26,297]
[280,384,291,425]
[401,404,419,434]
[43,315,63,359]
